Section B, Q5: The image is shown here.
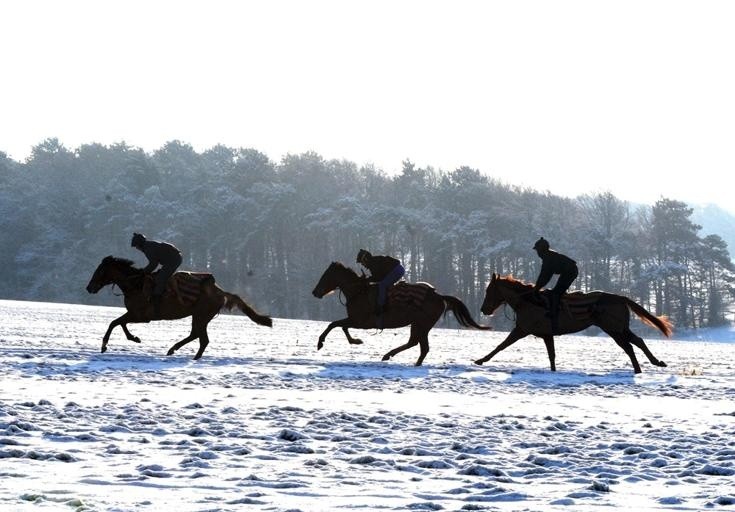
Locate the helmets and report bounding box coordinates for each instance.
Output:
[533,237,550,250]
[356,249,372,263]
[130,231,147,247]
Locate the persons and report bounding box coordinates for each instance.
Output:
[356,249,404,299]
[532,238,578,323]
[131,234,183,297]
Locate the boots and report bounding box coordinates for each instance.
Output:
[152,293,163,315]
[543,293,561,318]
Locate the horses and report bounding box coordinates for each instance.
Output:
[86,255,272,361]
[311,260,493,367]
[474,273,671,374]
